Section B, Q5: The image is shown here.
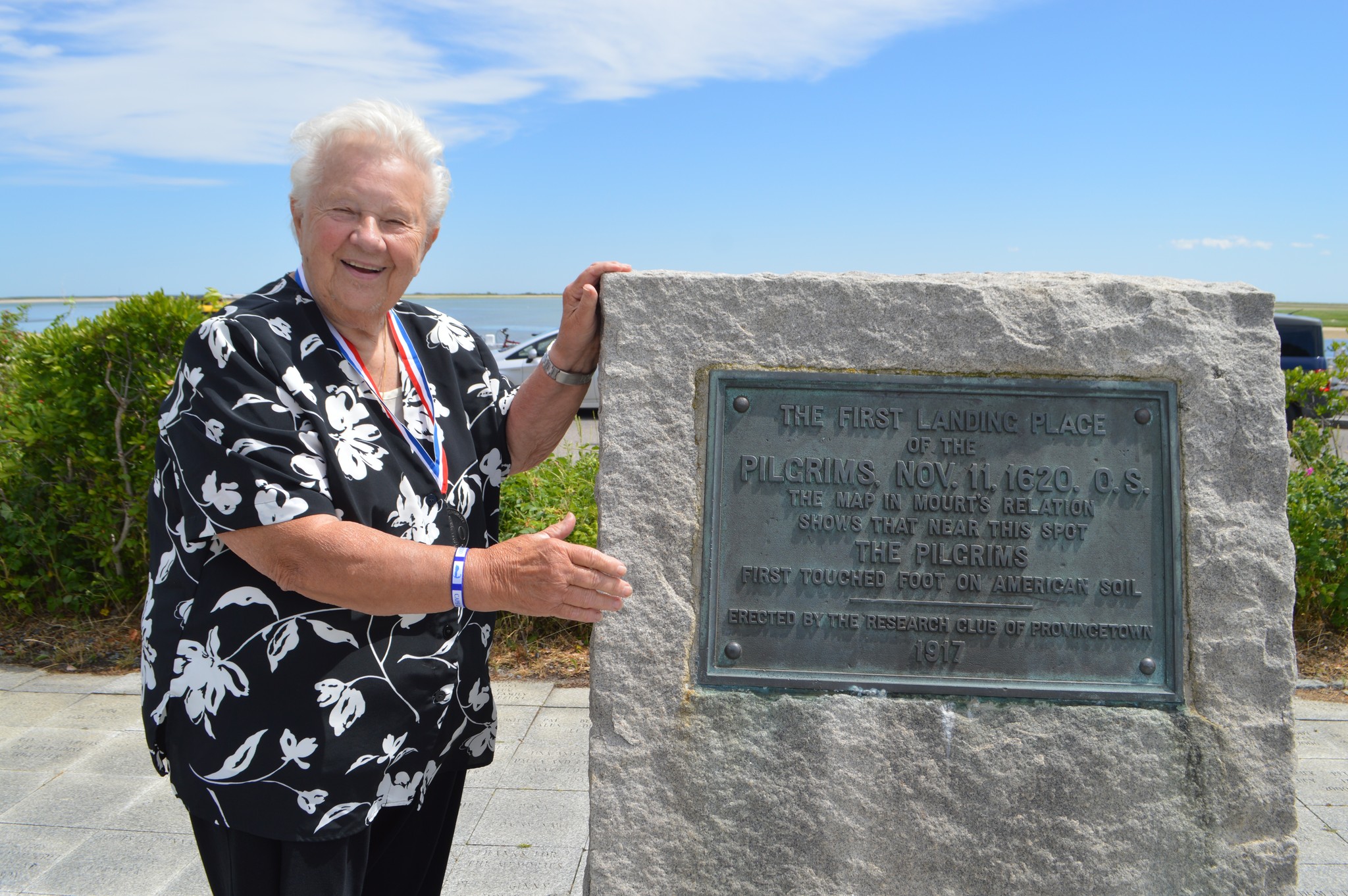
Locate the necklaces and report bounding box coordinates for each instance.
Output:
[376,313,386,401]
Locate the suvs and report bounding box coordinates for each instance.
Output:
[1270,309,1332,432]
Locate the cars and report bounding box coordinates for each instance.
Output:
[493,329,603,409]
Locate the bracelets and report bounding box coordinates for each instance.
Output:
[451,546,469,610]
[543,338,598,385]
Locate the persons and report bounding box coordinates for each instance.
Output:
[139,95,635,896]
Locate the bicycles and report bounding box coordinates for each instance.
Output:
[497,327,543,352]
